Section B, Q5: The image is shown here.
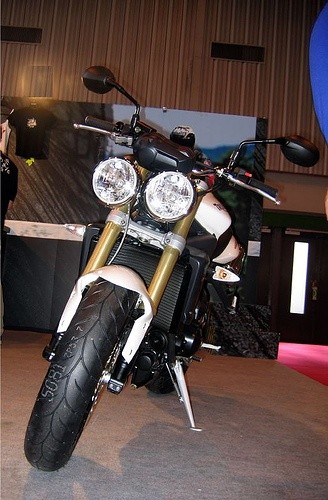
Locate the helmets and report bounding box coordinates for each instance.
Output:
[170,126,195,149]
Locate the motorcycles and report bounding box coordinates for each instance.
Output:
[24,64,318,474]
